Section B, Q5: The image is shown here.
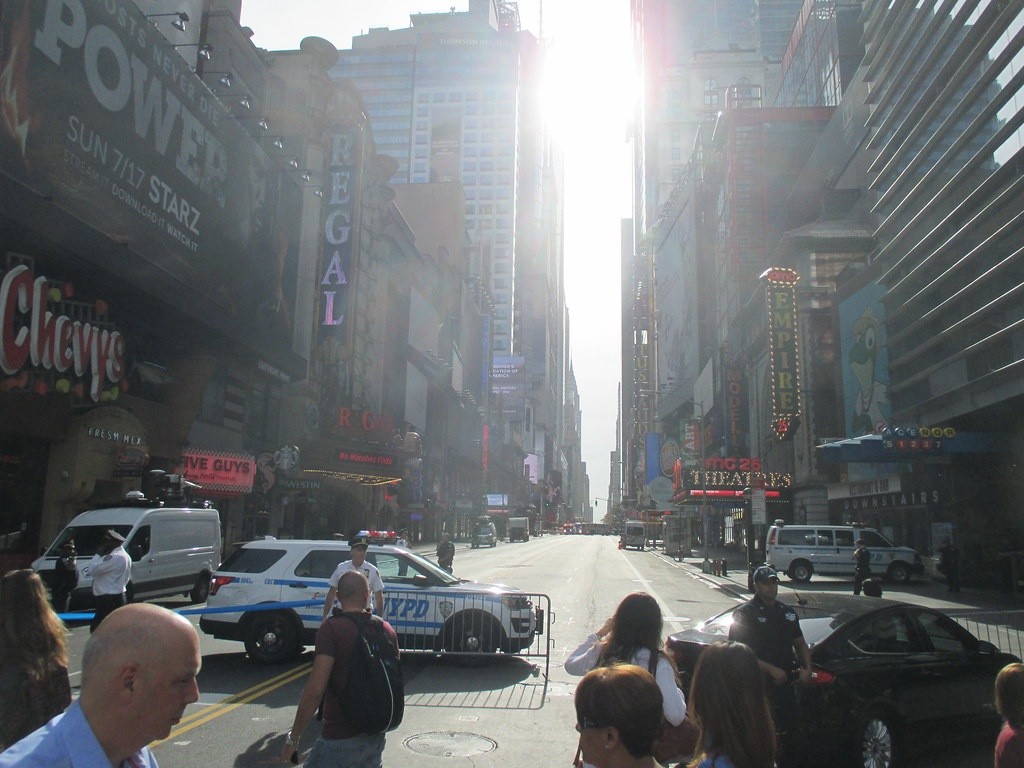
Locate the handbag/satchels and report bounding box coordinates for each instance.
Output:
[447,567,453,574]
[648,648,700,762]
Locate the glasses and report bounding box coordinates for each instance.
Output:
[576,714,613,733]
[353,546,367,552]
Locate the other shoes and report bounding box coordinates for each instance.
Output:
[317,712,323,721]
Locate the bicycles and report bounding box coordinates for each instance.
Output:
[674,546,685,563]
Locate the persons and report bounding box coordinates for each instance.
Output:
[283,536,400,768]
[88,530,132,633]
[55,537,78,613]
[564,593,687,768]
[436,533,455,574]
[853,540,884,597]
[993,663,1024,768]
[396,528,412,576]
[0,570,203,768]
[688,566,811,768]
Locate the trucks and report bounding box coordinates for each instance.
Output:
[507,517,529,543]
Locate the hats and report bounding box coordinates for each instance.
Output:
[753,565,780,584]
[60,538,75,549]
[400,528,408,534]
[348,535,369,547]
[104,529,125,543]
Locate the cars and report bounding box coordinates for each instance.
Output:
[471,522,498,548]
[200,531,544,667]
[668,591,1021,768]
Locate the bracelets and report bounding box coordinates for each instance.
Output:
[286,731,300,746]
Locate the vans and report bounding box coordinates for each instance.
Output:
[30,492,221,614]
[623,521,646,551]
[765,520,923,584]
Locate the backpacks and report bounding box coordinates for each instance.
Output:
[330,613,405,732]
